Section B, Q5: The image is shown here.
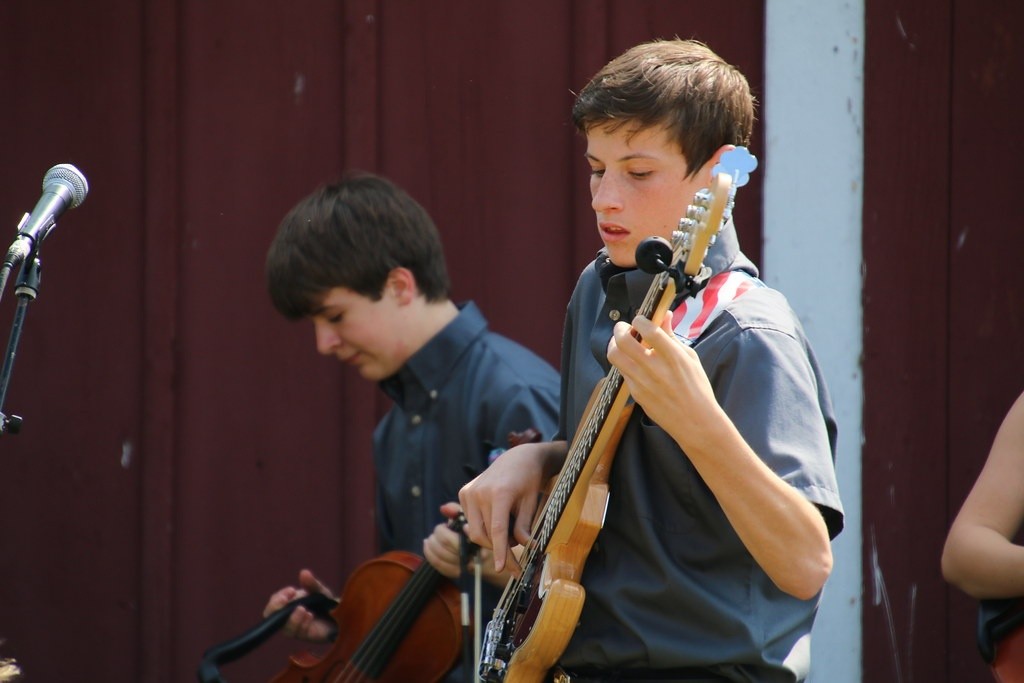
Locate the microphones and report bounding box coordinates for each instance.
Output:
[5,164,89,269]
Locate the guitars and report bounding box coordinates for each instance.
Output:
[480,145,759,683]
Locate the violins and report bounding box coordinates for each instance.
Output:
[269,424,546,683]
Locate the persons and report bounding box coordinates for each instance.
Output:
[258,173,564,683]
[458,41,844,683]
[940,382,1024,682]
[263,177,569,682]
